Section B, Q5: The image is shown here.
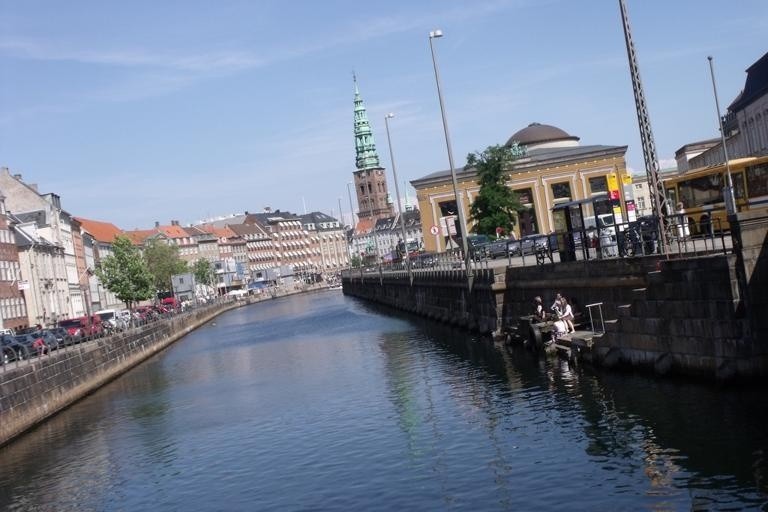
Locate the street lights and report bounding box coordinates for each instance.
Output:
[361,172,383,287]
[429,29,473,292]
[385,113,413,285]
[337,197,353,285]
[345,180,365,284]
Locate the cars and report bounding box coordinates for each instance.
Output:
[454,213,625,270]
[392,254,438,270]
[0,298,175,366]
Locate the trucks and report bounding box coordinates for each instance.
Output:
[390,238,421,258]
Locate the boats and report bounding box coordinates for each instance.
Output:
[329,286,342,291]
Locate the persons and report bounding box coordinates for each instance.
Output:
[32,336,48,356]
[673,202,690,248]
[532,293,575,344]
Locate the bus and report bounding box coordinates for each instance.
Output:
[663,155,768,233]
